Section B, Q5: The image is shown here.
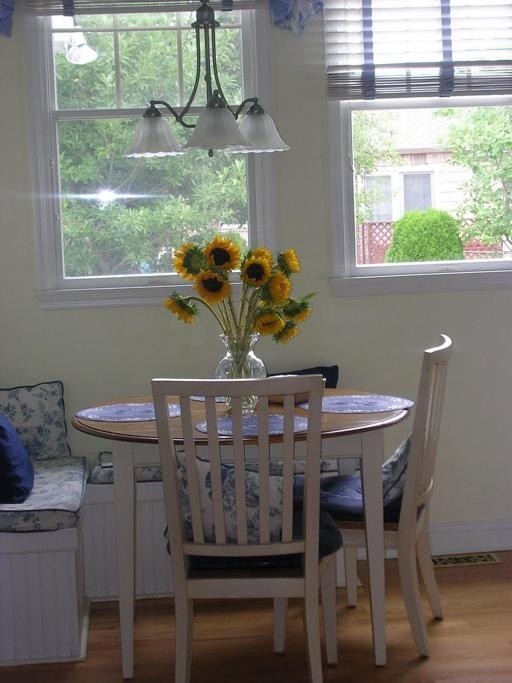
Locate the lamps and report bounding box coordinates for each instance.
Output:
[121,0,291,157]
[52,15,99,65]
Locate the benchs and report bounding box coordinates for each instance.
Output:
[80,480,362,602]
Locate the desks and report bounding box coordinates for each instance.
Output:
[72,390,410,682]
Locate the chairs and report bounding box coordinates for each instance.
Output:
[151,374,342,683]
[273,334,453,658]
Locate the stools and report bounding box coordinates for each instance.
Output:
[0,457,91,668]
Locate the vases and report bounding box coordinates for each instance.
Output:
[215,333,266,414]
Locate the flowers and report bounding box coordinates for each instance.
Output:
[162,233,321,345]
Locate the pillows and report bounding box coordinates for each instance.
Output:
[0,381,71,462]
[164,450,296,546]
[0,411,34,504]
[267,365,339,389]
[383,435,409,505]
